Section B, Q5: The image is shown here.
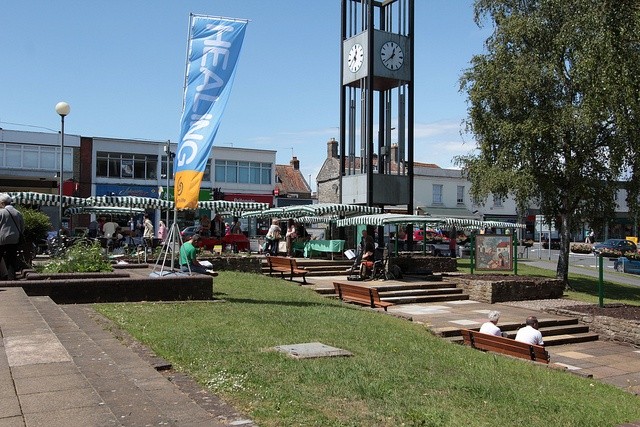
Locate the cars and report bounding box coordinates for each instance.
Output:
[405,230,457,258]
[181,226,211,238]
[592,239,636,255]
[614,257,640,273]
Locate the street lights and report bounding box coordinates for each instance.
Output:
[56,102,70,228]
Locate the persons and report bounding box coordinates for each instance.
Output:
[220,216,225,223]
[230,221,235,230]
[158,220,173,254]
[515,315,546,347]
[179,234,219,277]
[585,229,591,244]
[91,215,143,252]
[456,233,472,258]
[478,310,501,336]
[505,228,510,234]
[198,214,210,235]
[143,213,155,238]
[539,232,544,242]
[266,218,281,256]
[232,221,242,254]
[589,228,595,244]
[264,228,272,253]
[211,213,225,237]
[0,192,24,280]
[285,218,296,259]
[223,221,231,253]
[359,230,375,279]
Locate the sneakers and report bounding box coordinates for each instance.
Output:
[206,269,218,276]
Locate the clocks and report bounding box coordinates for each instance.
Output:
[348,43,364,72]
[380,41,404,71]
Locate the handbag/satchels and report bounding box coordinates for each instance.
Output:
[17,233,32,249]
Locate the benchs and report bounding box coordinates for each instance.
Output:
[266,256,309,284]
[461,328,550,364]
[333,282,395,311]
